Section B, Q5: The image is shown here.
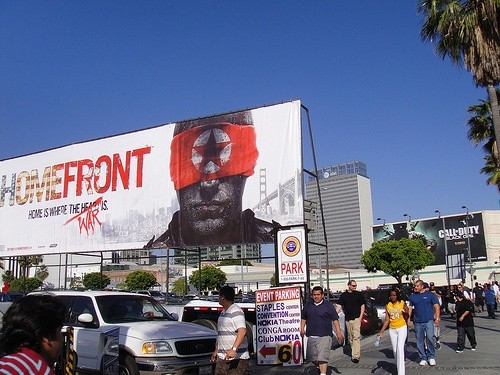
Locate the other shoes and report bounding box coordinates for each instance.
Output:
[470,345,478,351]
[418,358,428,367]
[316,366,320,374]
[353,357,360,363]
[455,348,464,354]
[429,357,437,366]
[436,342,442,351]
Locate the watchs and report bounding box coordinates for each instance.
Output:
[231,347,238,351]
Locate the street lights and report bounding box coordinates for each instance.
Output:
[435,210,450,289]
[461,206,475,305]
[316,186,328,289]
[344,271,351,281]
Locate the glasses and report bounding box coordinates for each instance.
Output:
[457,287,461,289]
[350,284,358,288]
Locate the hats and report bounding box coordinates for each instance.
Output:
[482,283,487,287]
[457,293,466,299]
[429,281,435,286]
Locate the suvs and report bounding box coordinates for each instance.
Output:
[25,289,219,375]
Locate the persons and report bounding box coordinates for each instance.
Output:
[455,292,478,353]
[378,288,409,375]
[300,286,344,375]
[443,281,500,321]
[336,280,365,363]
[407,280,442,366]
[211,286,250,375]
[0,295,69,375]
[151,111,282,247]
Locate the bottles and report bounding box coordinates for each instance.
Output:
[375,336,380,346]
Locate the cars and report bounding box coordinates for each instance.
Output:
[0,291,30,331]
[124,283,472,356]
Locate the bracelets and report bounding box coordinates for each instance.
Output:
[436,318,440,321]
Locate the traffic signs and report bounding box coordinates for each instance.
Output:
[254,286,304,366]
